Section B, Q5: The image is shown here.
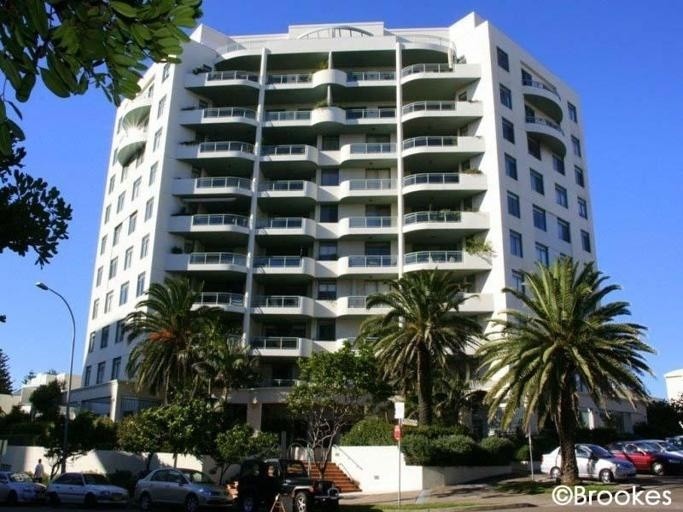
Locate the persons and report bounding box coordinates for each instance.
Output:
[32,458,44,482]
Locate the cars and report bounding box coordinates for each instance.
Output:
[540,435,683,484]
[0,467,234,512]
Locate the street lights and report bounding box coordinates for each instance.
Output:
[34,281,76,475]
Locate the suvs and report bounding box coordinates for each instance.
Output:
[238,458,344,512]
